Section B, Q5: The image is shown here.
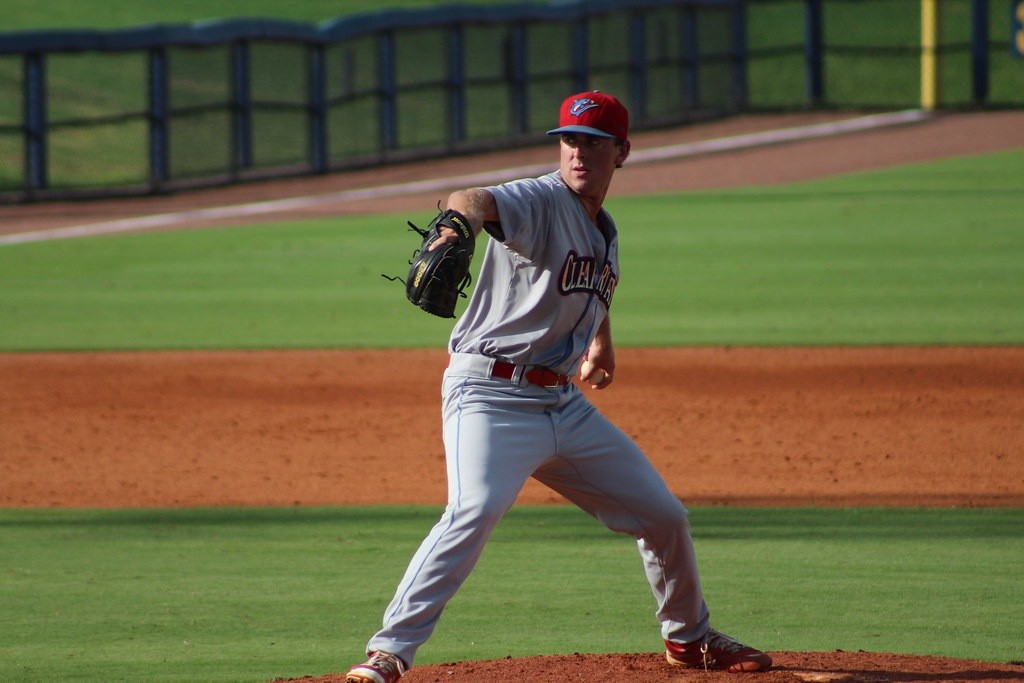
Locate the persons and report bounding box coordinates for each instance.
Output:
[346,89,772,683]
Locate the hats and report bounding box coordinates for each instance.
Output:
[546,88,627,141]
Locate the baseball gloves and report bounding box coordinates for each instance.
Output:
[403,208,476,318]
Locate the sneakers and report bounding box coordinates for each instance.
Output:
[347,648,405,683]
[665,628,772,671]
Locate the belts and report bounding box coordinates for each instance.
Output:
[492,362,571,387]
